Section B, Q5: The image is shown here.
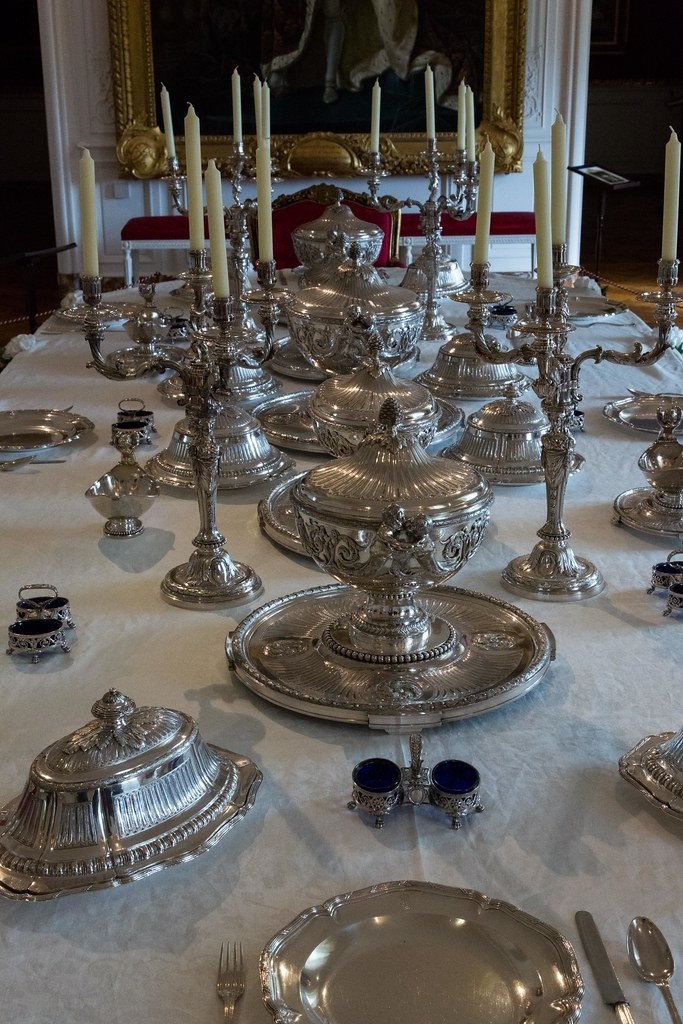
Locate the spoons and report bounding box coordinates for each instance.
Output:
[627,387,683,396]
[626,917,683,1024]
[1,455,37,471]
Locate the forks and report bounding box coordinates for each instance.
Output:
[572,321,636,329]
[217,942,246,1024]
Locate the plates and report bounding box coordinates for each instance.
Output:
[1,409,95,452]
[259,881,585,1022]
[53,301,148,325]
[565,295,629,321]
[602,396,683,435]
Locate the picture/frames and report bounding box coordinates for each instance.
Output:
[107,0,528,175]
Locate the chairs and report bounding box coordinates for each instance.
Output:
[248,183,401,269]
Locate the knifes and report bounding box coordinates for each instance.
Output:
[30,459,67,464]
[574,911,635,1024]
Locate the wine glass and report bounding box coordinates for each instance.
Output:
[83,449,160,538]
[122,307,172,359]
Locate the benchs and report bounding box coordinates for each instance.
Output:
[121,212,537,290]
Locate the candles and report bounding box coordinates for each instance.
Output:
[371,77,381,154]
[254,72,274,261]
[184,102,204,252]
[458,76,466,149]
[661,126,681,260]
[533,144,553,289]
[474,134,495,263]
[232,65,242,144]
[551,107,566,244]
[466,84,475,161]
[425,62,436,138]
[79,142,99,277]
[205,157,229,299]
[160,81,176,158]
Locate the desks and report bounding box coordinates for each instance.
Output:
[0,275,683,1024]
